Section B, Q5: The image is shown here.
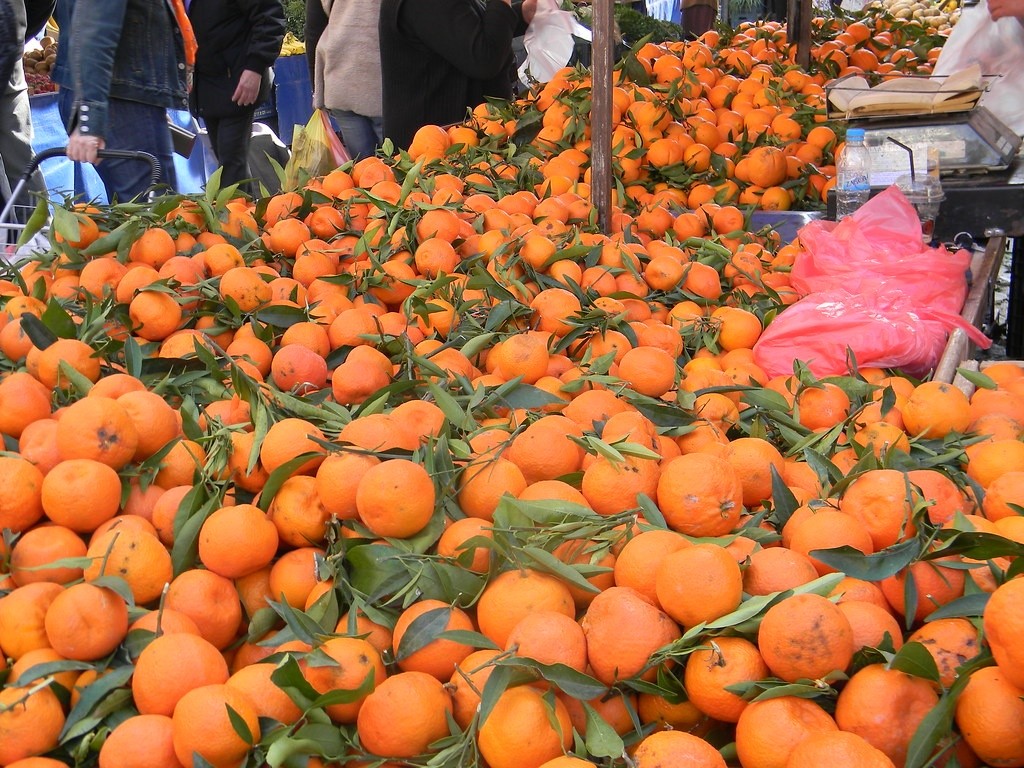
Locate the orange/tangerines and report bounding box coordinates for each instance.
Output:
[0,17,1024,768]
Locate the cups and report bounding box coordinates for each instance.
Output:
[888,171,946,248]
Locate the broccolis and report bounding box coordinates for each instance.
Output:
[576,3,683,44]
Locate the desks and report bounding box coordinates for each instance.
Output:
[826,144,1024,362]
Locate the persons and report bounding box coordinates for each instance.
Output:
[52,0,196,212]
[987,0,1024,29]
[183,0,288,192]
[1,0,55,246]
[307,0,571,162]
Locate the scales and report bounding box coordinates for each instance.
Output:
[845,104,1022,176]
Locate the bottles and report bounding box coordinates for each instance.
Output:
[834,127,872,224]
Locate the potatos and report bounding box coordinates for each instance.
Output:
[868,0,961,31]
